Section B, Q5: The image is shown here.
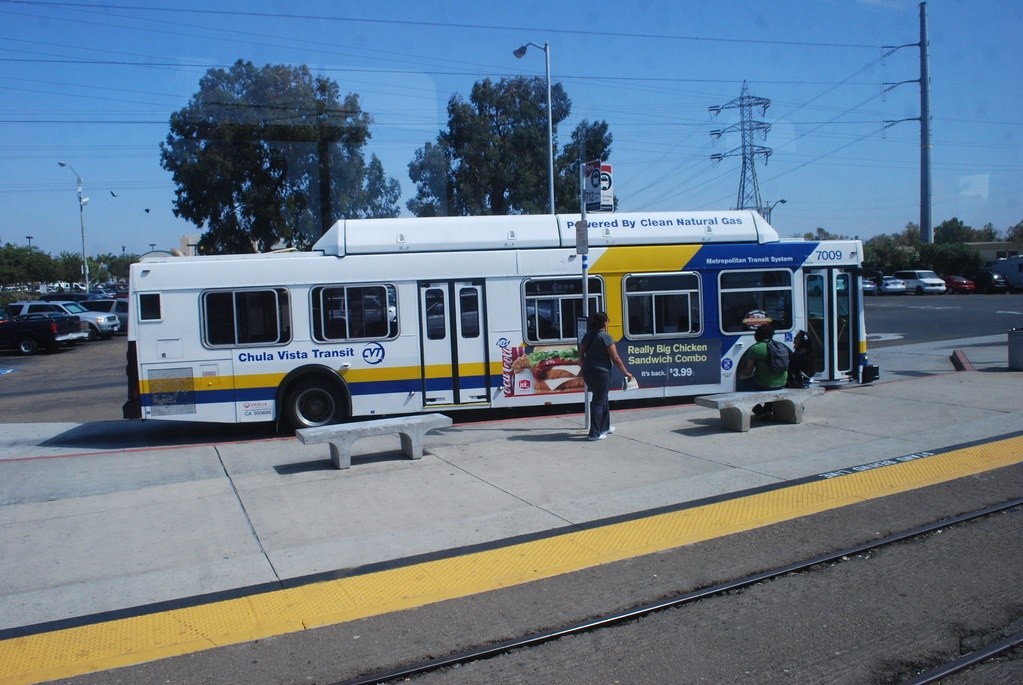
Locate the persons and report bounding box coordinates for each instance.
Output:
[874,271,884,296]
[789,330,818,389]
[738,324,790,391]
[578,312,632,440]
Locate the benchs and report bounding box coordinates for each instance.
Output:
[694,385,825,433]
[295,413,452,471]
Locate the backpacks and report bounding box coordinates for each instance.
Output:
[756,340,790,372]
[798,351,816,381]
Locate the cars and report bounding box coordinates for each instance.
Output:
[862,277,875,295]
[941,275,975,295]
[38,293,102,301]
[877,276,906,296]
[113,291,128,298]
[807,275,844,296]
[78,298,129,330]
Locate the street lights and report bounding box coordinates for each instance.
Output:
[769,199,787,225]
[513,42,554,214]
[58,160,89,291]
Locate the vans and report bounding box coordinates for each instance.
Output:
[892,270,946,295]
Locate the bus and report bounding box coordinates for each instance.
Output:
[122,208,880,436]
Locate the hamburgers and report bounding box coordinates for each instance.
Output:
[511,345,585,392]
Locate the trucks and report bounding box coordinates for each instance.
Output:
[992,255,1023,293]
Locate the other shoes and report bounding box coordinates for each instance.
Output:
[757,407,772,418]
[588,433,607,440]
[601,425,616,434]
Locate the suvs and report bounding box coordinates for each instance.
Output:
[8,300,120,342]
[87,289,116,299]
[973,271,1008,294]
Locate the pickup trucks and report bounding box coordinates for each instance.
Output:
[0,303,92,355]
